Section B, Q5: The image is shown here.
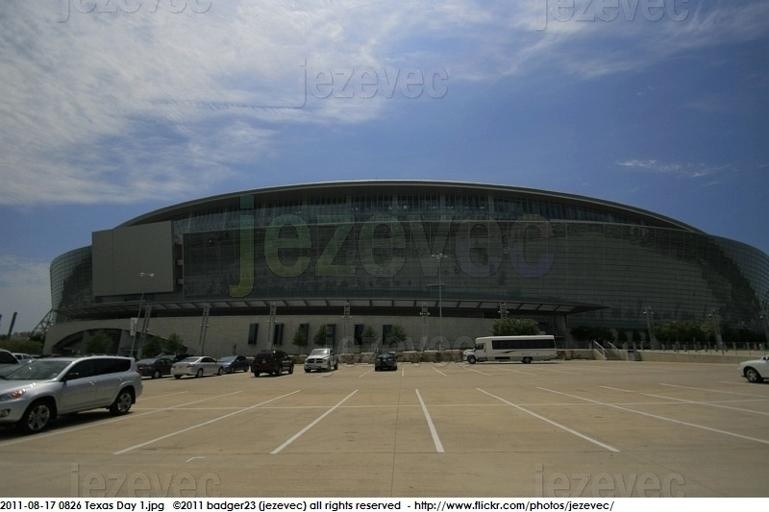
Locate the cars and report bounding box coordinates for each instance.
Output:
[738,354,769,383]
[304,349,338,372]
[375,352,397,371]
[0,348,293,434]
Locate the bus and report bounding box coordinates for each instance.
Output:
[463,335,555,364]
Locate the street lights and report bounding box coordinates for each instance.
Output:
[430,253,448,350]
[129,271,154,357]
[643,307,656,350]
